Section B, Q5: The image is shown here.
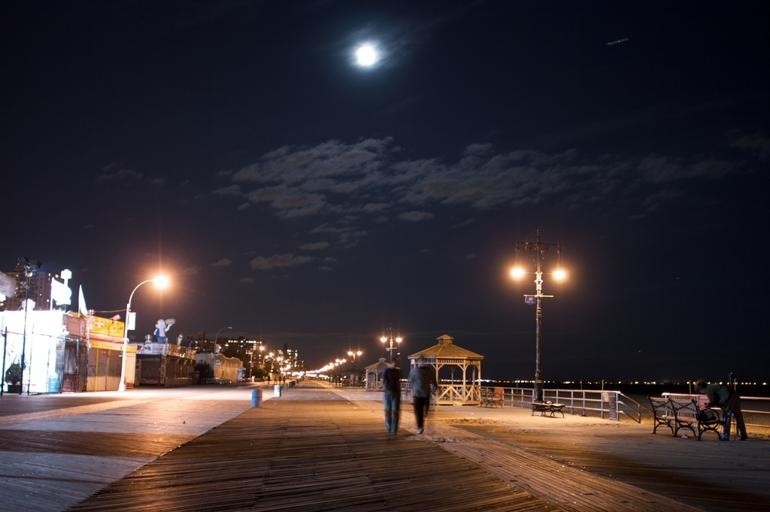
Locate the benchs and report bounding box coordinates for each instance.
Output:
[531,403,566,418]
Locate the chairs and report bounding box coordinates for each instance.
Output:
[646,394,730,442]
[479,389,504,409]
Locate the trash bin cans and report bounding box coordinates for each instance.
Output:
[252,389,263,408]
[273,385,283,397]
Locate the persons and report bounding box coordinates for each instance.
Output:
[382,358,402,435]
[405,355,440,435]
[695,380,750,444]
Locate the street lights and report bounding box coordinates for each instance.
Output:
[508,228,568,403]
[335,344,364,382]
[117,276,171,390]
[20,265,32,394]
[212,327,232,378]
[380,328,403,363]
